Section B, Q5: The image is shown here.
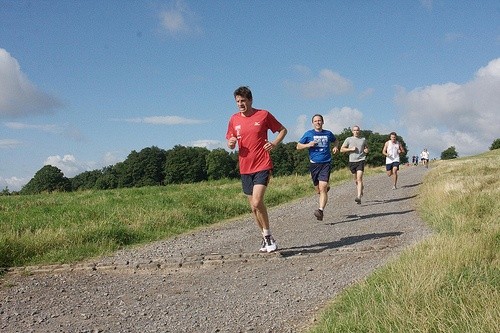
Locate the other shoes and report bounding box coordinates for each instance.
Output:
[355,197,361,204]
[397,165,399,170]
[361,186,363,196]
[392,185,396,190]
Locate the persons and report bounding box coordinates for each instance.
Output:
[340,126,369,204]
[383,132,405,190]
[412,148,430,168]
[296,114,340,221]
[226,87,287,253]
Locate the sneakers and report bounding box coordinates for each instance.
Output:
[314,209,323,221]
[326,185,330,192]
[263,234,277,253]
[259,238,267,252]
